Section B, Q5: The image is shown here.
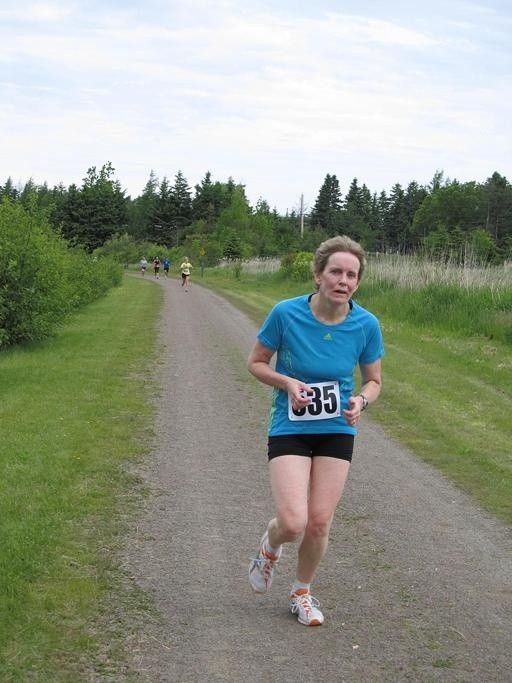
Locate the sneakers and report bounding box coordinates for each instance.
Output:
[286,586,325,627]
[246,527,283,595]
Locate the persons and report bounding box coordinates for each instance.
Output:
[245,233,385,626]
[180,256,193,292]
[151,255,161,280]
[161,255,172,279]
[138,255,148,278]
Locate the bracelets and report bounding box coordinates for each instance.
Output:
[359,395,370,411]
